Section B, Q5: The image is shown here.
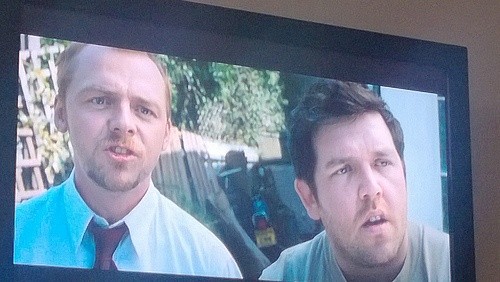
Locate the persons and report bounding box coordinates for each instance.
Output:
[257,77,451,282]
[11,42,243,279]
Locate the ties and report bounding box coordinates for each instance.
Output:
[88,219,128,272]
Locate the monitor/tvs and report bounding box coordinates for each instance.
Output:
[0,0,475,281]
